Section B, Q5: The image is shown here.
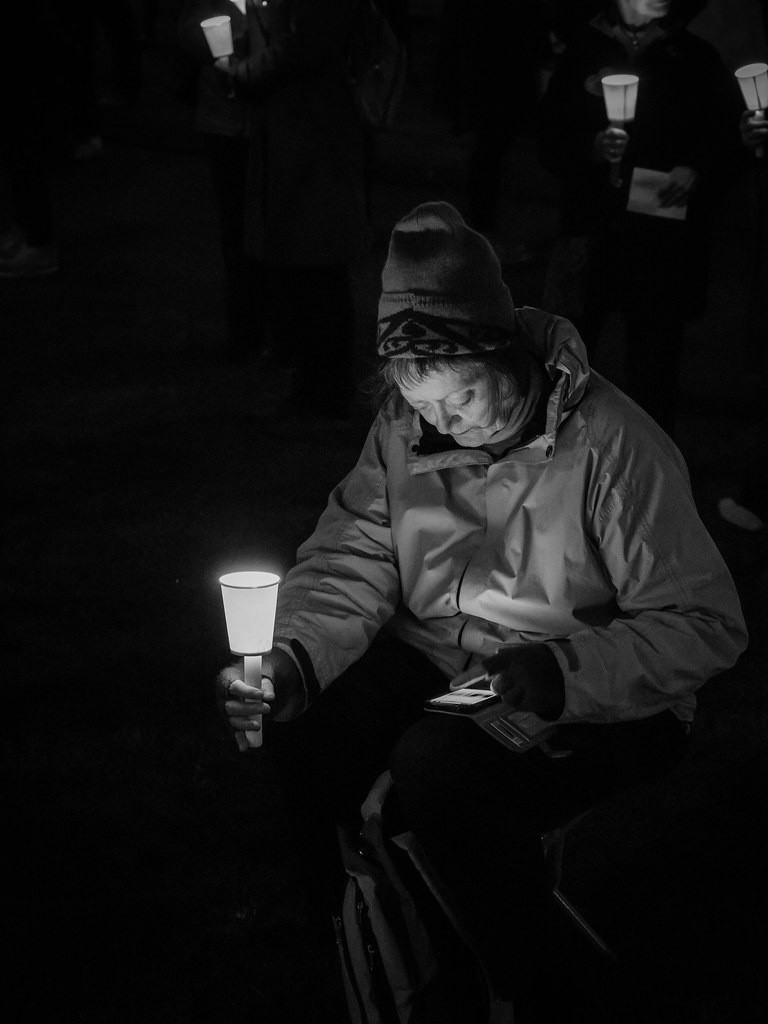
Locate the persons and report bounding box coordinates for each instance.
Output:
[546,1,768,211]
[207,197,748,1024]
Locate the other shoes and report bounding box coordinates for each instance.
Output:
[0,224,59,281]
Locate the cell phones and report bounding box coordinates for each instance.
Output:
[422,675,500,714]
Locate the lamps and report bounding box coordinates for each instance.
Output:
[199,16,235,99]
[217,570,283,749]
[736,63,768,158]
[602,73,641,190]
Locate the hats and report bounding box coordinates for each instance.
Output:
[377,201,514,361]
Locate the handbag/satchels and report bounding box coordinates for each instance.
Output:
[332,772,496,1024]
